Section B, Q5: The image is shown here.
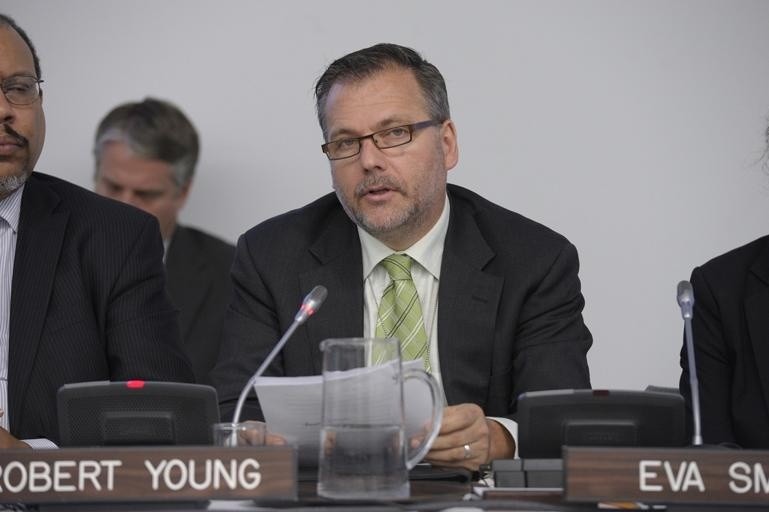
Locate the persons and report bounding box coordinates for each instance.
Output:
[0,15,196,453]
[209,41,596,473]
[92,95,237,368]
[676,233,769,449]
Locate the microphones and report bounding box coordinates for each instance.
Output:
[232,285,329,447]
[675,280,704,447]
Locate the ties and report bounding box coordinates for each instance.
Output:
[371,254,430,373]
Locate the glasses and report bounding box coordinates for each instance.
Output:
[0,74,44,105]
[321,120,442,160]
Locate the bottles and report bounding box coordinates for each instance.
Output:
[315,339,445,502]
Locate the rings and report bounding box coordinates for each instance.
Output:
[464,443,471,460]
